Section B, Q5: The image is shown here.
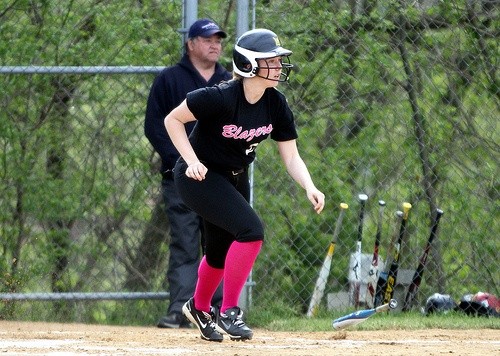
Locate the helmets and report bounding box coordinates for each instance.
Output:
[460,292,500,317]
[232,28,294,79]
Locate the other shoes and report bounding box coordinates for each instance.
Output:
[156,309,184,329]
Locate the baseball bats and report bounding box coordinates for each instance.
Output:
[374,202,412,308]
[350,194,368,311]
[332,299,398,331]
[366,200,386,309]
[306,202,348,319]
[402,208,444,312]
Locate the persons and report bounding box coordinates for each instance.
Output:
[144,19,233,328]
[163,28,325,342]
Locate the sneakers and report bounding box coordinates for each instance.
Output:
[215,306,254,342]
[181,295,224,342]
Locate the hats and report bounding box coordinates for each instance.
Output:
[187,18,228,39]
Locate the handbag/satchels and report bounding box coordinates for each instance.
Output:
[423,291,455,315]
